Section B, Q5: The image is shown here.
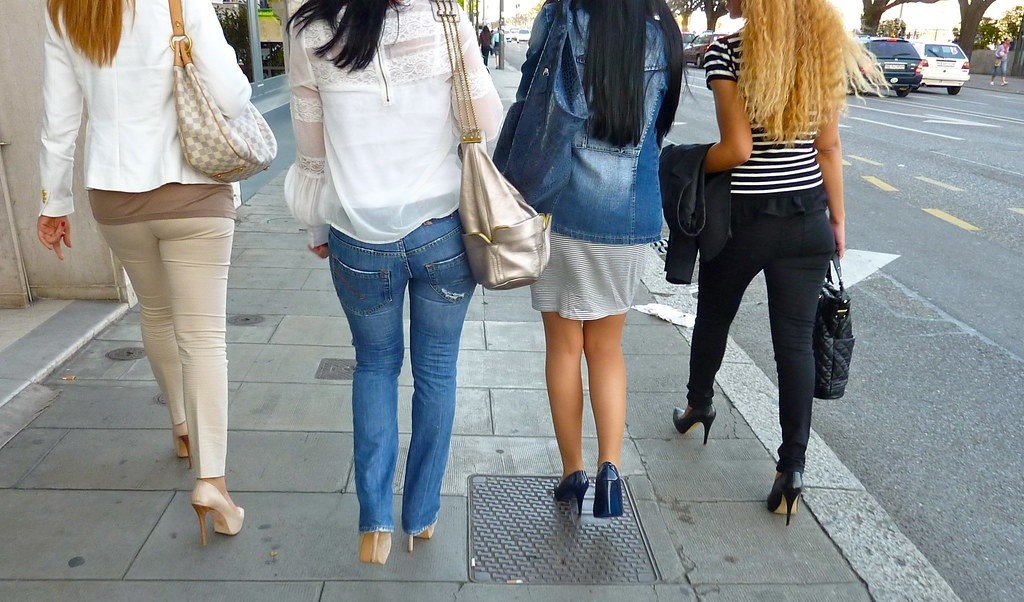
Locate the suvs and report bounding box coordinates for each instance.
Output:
[684,30,730,68]
[845,35,924,97]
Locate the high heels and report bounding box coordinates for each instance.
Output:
[554,470,589,517]
[407,516,437,552]
[191,479,245,546]
[593,462,624,517]
[359,526,391,565]
[673,402,716,444]
[173,422,193,469]
[766,466,803,526]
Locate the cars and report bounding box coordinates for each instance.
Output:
[502,26,530,43]
[681,32,696,48]
[908,39,971,95]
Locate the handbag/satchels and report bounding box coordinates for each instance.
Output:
[168,0,278,181]
[459,140,553,290]
[811,249,854,401]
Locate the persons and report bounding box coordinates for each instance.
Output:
[660,0,892,540]
[38,0,278,546]
[990,38,1010,87]
[492,1,684,523]
[278,1,507,568]
[478,25,507,70]
